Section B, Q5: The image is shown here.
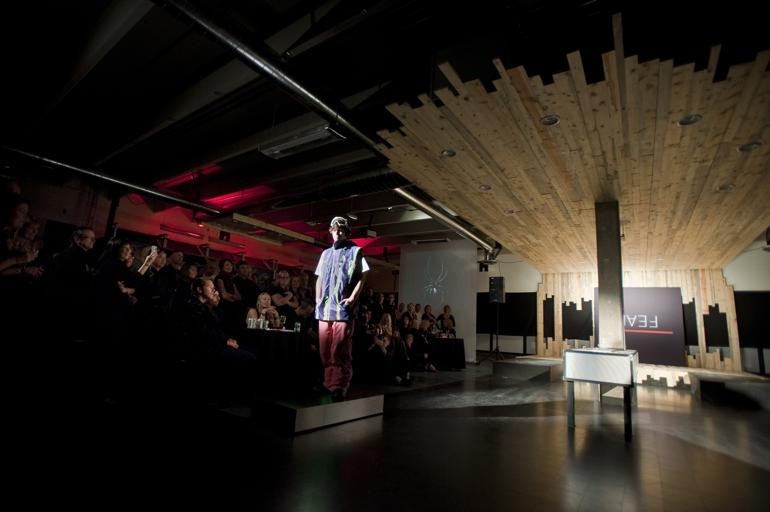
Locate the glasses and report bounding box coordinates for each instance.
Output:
[330,217,350,229]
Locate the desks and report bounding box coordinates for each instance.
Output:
[562,347,641,444]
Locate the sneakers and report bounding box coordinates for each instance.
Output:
[313,385,346,402]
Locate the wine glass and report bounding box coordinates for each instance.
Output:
[279,316,286,330]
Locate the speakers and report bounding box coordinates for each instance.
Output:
[488,276,505,304]
